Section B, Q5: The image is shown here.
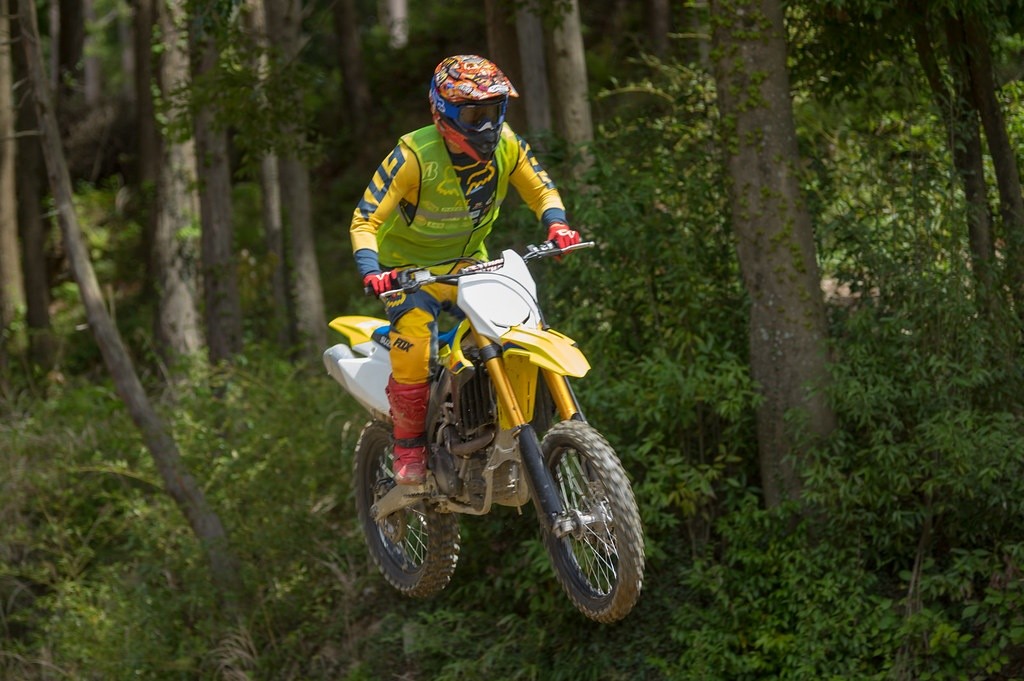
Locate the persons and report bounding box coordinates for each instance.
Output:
[350,55,583,498]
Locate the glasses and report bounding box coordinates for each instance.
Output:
[431,75,508,133]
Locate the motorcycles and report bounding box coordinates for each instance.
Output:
[320,234,647,627]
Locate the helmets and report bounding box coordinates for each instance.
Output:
[428,54,520,166]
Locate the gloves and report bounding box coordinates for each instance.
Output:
[546,224,581,262]
[363,268,402,300]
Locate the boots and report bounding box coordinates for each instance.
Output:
[385,372,431,488]
